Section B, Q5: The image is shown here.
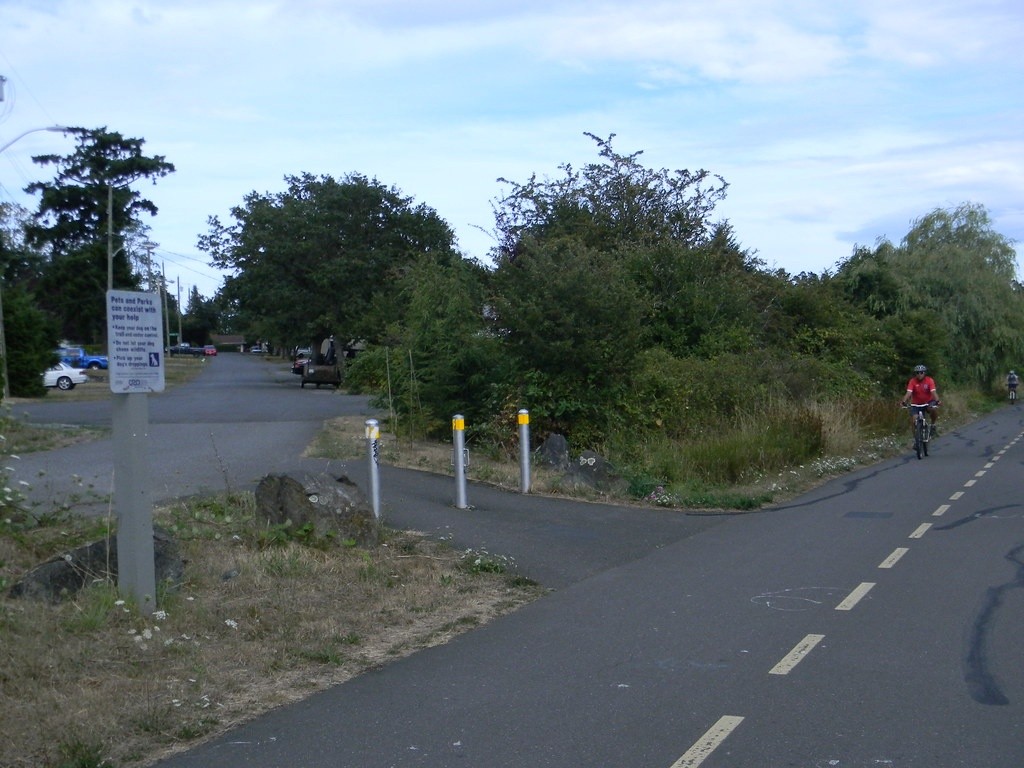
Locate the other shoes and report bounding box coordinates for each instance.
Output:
[912,441,917,450]
[930,425,937,436]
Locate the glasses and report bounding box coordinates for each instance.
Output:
[917,371,925,375]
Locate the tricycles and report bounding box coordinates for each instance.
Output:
[300,365,343,388]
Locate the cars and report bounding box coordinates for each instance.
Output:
[292,345,312,374]
[203,344,217,357]
[41,360,88,390]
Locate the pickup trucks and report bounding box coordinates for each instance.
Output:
[49,347,109,371]
[165,342,206,357]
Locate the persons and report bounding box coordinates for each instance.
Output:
[899,365,941,450]
[1005,370,1019,399]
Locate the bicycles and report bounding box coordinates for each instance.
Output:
[1005,381,1021,404]
[900,400,939,459]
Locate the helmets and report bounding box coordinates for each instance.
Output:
[914,364,928,372]
[1009,370,1014,375]
